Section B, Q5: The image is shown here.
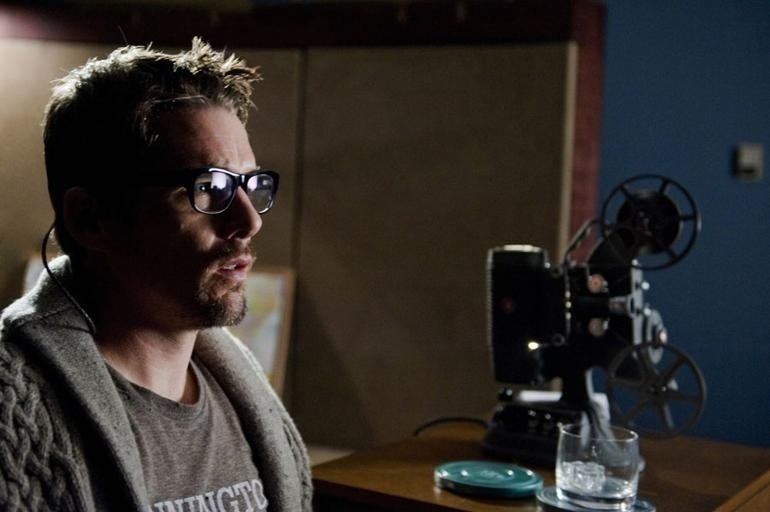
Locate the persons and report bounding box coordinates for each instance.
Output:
[0,37,314,512]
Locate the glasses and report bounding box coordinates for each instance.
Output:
[73,166,280,217]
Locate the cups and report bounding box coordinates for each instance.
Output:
[555,422,640,510]
